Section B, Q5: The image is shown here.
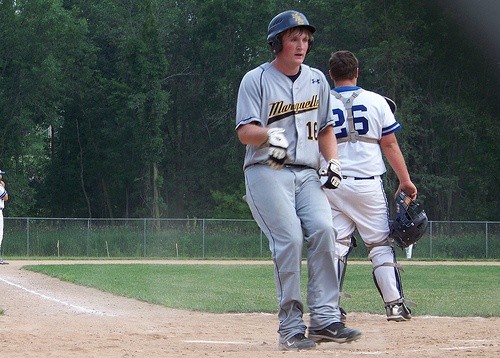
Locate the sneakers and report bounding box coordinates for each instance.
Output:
[386,303,411,322]
[308,322,361,343]
[278,333,316,351]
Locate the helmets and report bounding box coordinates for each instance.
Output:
[389,189,428,251]
[267,10,316,55]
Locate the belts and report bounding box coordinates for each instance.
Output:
[343,176,374,180]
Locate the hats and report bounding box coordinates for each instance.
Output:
[0,170,5,174]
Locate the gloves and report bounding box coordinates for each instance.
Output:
[266,127,291,168]
[318,159,342,190]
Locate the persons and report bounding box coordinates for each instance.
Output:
[0,170,8,264]
[235,11,362,351]
[319,50,429,322]
